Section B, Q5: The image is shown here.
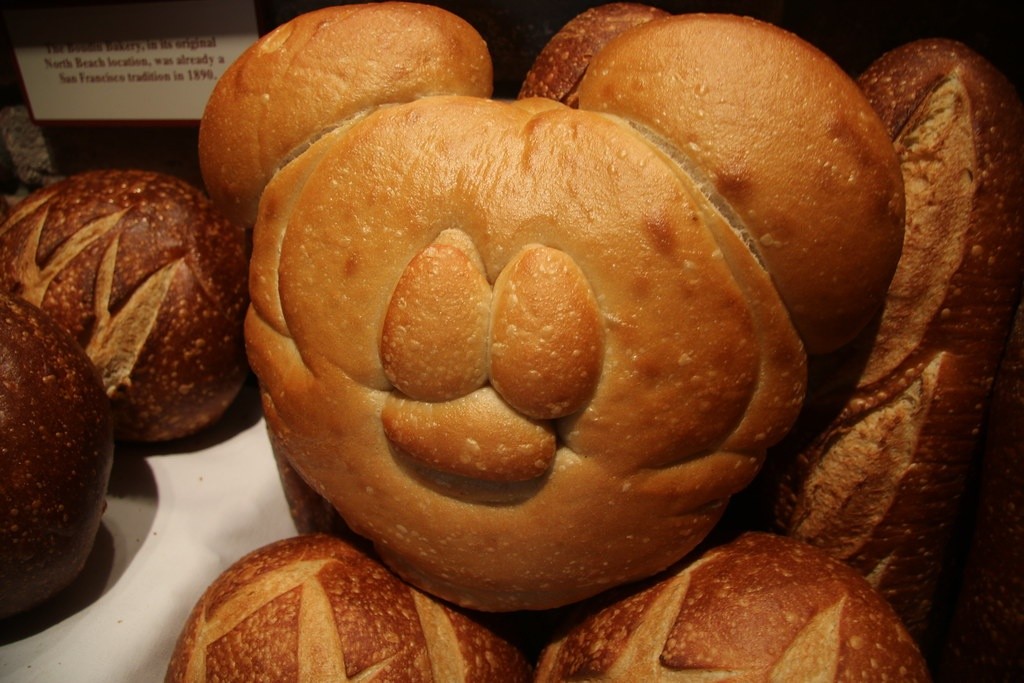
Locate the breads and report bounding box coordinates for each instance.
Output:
[0,0,1024,683]
